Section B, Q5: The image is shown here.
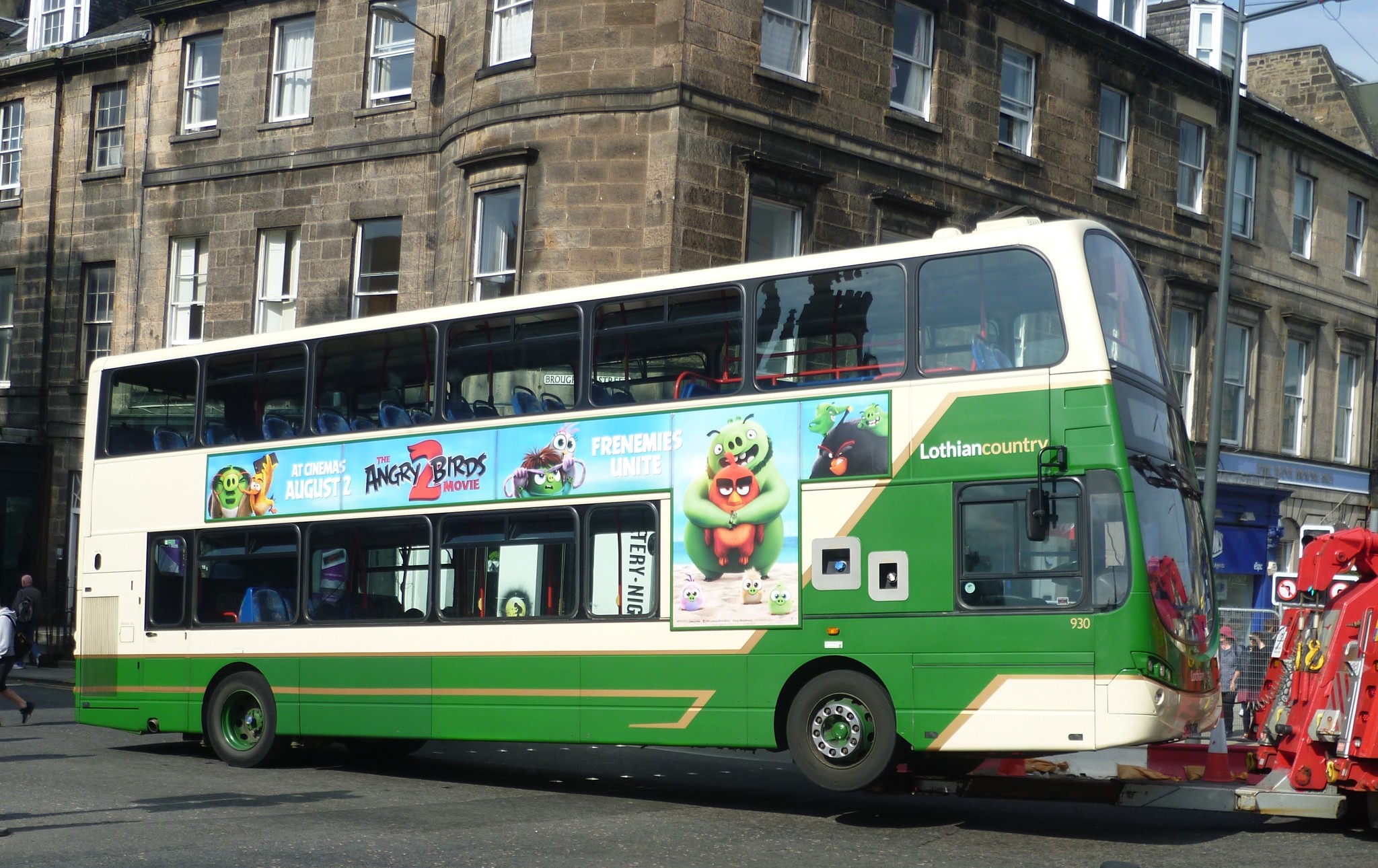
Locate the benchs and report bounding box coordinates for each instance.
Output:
[109,427,153,455]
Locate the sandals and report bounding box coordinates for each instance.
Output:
[1241,732,1248,739]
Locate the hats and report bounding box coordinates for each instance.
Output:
[1221,626,1237,640]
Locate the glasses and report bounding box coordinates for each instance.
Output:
[1249,638,1256,642]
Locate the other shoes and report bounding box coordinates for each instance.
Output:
[1239,709,1243,716]
[36,654,43,668]
[12,664,25,669]
[1226,730,1232,738]
[20,702,36,724]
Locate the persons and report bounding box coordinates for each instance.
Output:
[0,595,36,727]
[1229,631,1270,740]
[1218,626,1243,735]
[10,574,44,669]
[1238,619,1276,717]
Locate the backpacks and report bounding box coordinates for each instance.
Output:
[19,589,36,624]
[3,614,28,660]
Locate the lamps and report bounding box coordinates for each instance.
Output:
[1240,512,1256,521]
[370,3,446,74]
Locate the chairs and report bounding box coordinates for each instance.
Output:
[154,334,1013,451]
[236,586,295,623]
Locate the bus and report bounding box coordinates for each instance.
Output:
[77,217,1225,795]
